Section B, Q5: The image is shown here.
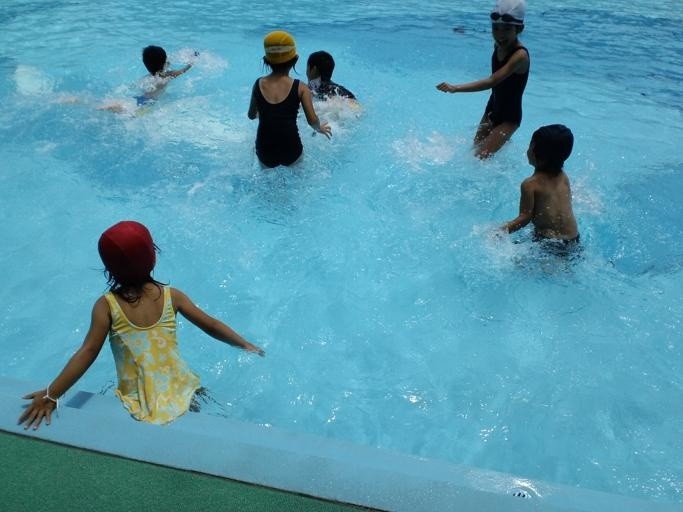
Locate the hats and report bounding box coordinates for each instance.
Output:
[491,0,524,25]
[263,31,297,61]
[98,220,156,274]
[530,124,574,161]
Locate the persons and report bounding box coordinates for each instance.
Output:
[294,49,359,113]
[15,218,265,434]
[89,42,201,115]
[434,1,531,161]
[491,123,581,258]
[244,28,332,174]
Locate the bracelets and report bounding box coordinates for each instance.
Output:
[40,386,66,416]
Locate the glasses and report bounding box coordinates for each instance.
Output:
[491,12,523,24]
